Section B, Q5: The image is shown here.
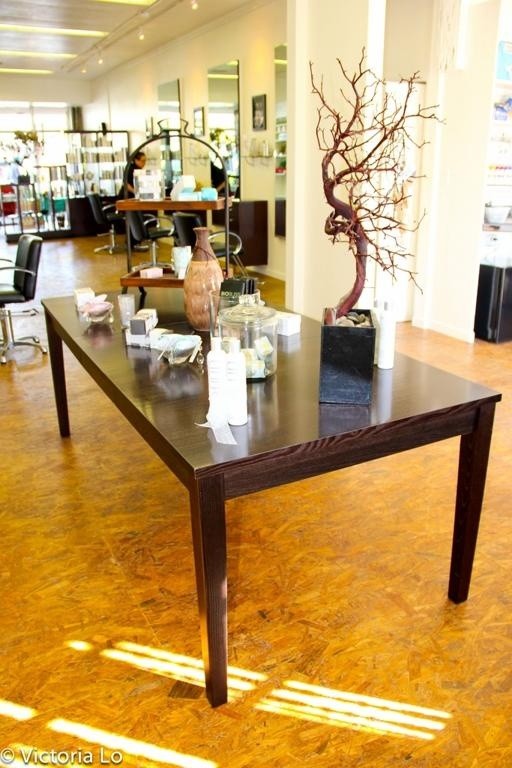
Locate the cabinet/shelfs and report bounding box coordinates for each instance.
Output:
[1,130,131,243]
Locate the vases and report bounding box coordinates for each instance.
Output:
[485,206,511,227]
[319,308,378,403]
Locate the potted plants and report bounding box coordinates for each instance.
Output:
[12,130,44,183]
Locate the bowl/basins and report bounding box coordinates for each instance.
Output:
[75,301,113,324]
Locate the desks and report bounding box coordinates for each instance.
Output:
[41,285,502,708]
[117,195,232,269]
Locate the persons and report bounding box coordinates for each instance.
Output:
[253,102,265,129]
[211,161,225,193]
[118,153,147,250]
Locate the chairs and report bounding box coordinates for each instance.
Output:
[173,212,258,283]
[127,209,175,271]
[0,235,47,365]
[85,195,124,254]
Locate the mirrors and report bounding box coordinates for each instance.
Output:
[207,62,239,199]
[275,45,288,236]
[157,78,183,186]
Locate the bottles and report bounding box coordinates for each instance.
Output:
[248,136,272,157]
[182,225,229,331]
[207,337,227,416]
[371,299,397,369]
[224,337,248,424]
[487,150,512,178]
[64,136,125,196]
[216,292,278,379]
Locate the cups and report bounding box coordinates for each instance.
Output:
[116,292,134,328]
[171,246,192,276]
[207,288,261,348]
